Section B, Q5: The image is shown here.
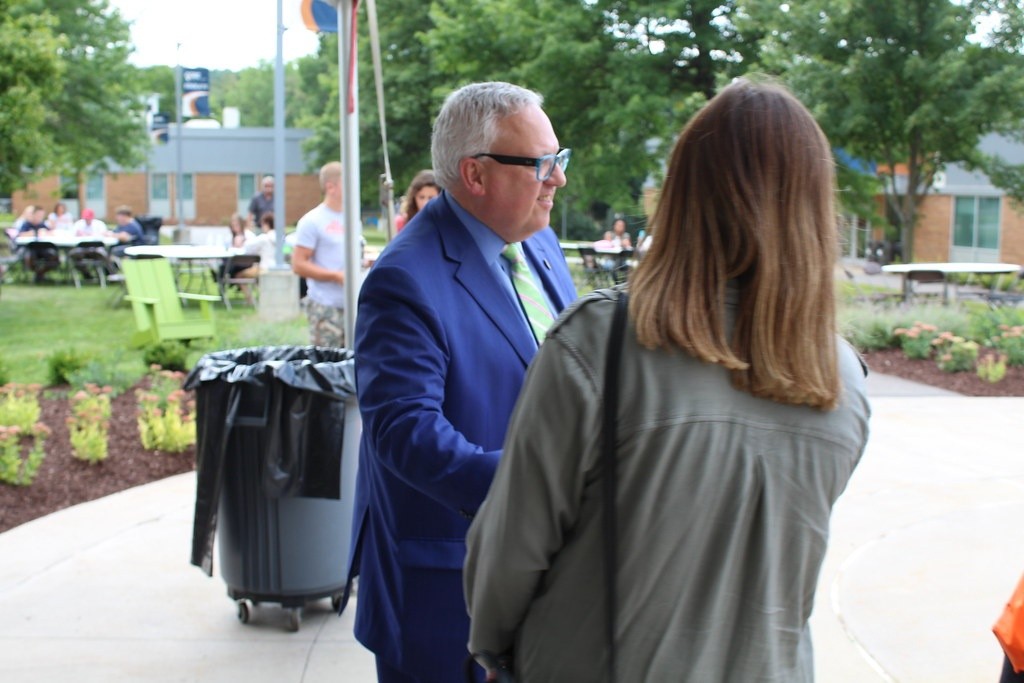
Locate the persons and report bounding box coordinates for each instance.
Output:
[990,571,1024,683]
[16,203,72,282]
[394,169,444,236]
[604,219,631,271]
[246,176,275,235]
[235,213,276,305]
[339,81,578,683]
[463,80,870,683]
[291,163,377,348]
[211,213,259,294]
[69,207,109,282]
[102,204,144,268]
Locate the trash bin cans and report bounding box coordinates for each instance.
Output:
[201,346,363,632]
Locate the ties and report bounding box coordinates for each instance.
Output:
[503,242,557,347]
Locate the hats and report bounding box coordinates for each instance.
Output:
[79,208,94,220]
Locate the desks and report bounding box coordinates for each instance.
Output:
[125,245,244,311]
[16,235,120,247]
[881,262,1023,306]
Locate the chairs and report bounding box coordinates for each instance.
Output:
[842,265,1024,316]
[578,247,640,291]
[0,218,261,348]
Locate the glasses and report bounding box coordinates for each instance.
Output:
[468,147,573,182]
